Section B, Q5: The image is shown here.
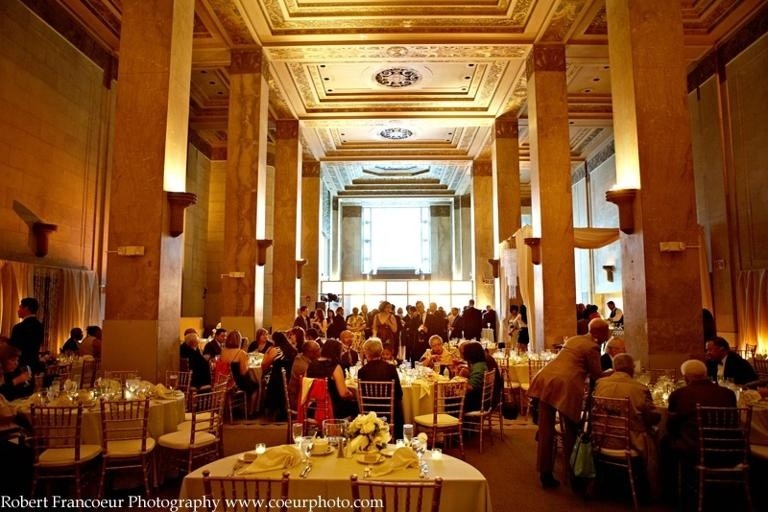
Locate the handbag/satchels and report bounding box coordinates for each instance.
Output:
[501,402,519,419]
[569,432,596,479]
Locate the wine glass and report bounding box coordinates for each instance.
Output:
[38,370,179,407]
[449,341,454,353]
[488,323,490,329]
[396,360,440,385]
[256,422,442,479]
[20,365,32,386]
[349,365,362,384]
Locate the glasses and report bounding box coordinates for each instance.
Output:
[600,329,610,334]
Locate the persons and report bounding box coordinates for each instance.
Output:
[2,299,768,510]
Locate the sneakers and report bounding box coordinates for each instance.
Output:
[541,474,560,489]
[569,476,589,491]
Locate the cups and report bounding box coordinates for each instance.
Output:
[639,373,675,407]
[716,376,744,403]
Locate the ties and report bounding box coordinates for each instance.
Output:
[419,315,425,341]
[343,346,354,366]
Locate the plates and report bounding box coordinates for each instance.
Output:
[238,451,257,462]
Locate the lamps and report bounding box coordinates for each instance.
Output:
[605,182,638,234]
